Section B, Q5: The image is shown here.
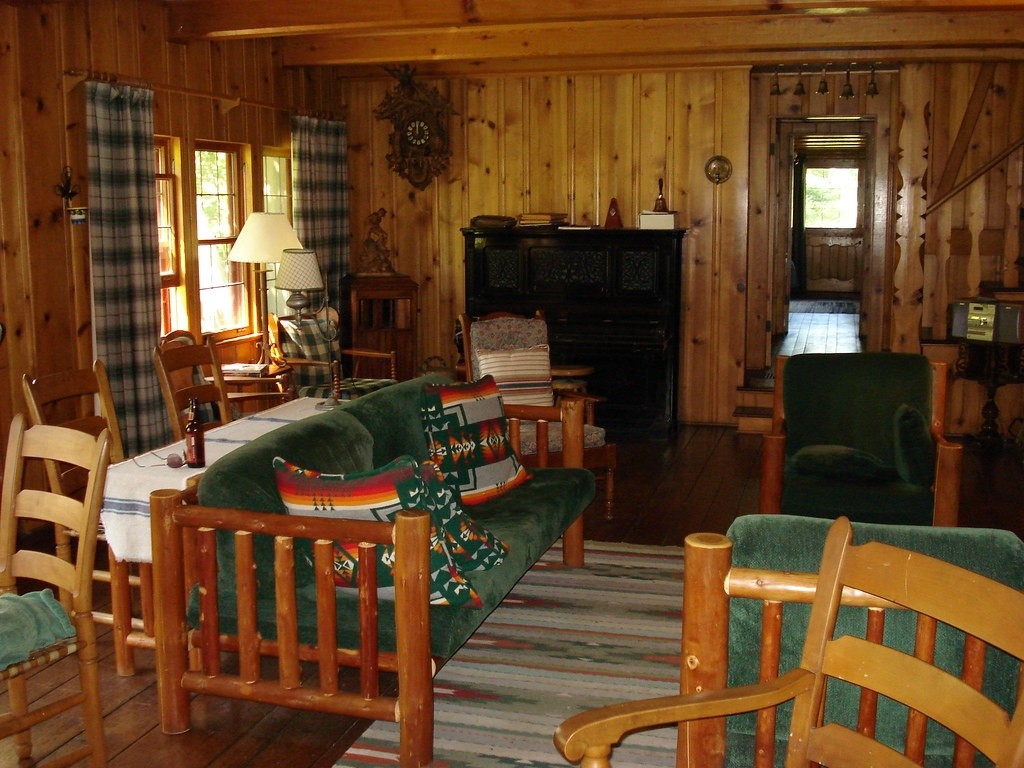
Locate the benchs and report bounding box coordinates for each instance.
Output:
[142,366,592,768]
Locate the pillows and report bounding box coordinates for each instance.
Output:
[418,459,514,571]
[790,445,887,487]
[265,452,484,617]
[416,372,531,501]
[894,404,935,483]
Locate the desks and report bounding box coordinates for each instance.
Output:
[921,337,1024,456]
[350,273,420,382]
[97,394,363,680]
[215,363,297,406]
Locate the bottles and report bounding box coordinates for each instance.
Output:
[185,398,205,468]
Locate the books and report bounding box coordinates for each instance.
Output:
[518,212,569,227]
[221,363,269,378]
[557,225,600,229]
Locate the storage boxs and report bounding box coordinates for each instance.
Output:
[639,211,681,230]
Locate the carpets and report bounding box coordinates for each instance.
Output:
[330,538,686,768]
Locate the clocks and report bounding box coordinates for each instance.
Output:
[372,64,462,191]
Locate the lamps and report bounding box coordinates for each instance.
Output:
[227,210,304,364]
[275,248,339,360]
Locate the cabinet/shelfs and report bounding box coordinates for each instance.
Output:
[462,226,689,448]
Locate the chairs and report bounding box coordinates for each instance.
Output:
[554,511,1024,768]
[679,510,1024,768]
[274,312,403,400]
[456,309,620,526]
[760,345,968,527]
[17,359,142,634]
[0,412,117,768]
[152,329,235,445]
